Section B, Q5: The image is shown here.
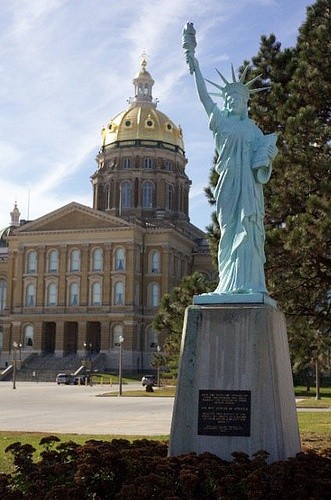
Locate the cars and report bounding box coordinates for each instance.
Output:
[56,373,70,385]
[142,375,156,386]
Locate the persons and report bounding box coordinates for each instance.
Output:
[188,54,281,296]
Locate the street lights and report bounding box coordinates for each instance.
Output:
[156,345,161,387]
[12,341,18,389]
[119,335,124,396]
